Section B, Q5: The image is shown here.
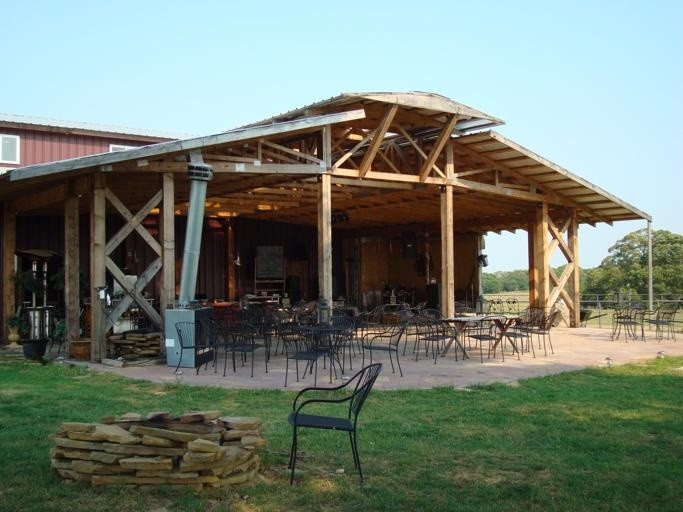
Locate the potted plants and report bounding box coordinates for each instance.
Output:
[6,271,67,362]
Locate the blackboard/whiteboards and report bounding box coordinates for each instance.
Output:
[256,246,284,278]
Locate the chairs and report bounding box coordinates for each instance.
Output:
[606,298,682,344]
[286,362,382,486]
[172,294,561,388]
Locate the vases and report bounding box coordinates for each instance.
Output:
[70,340,91,362]
